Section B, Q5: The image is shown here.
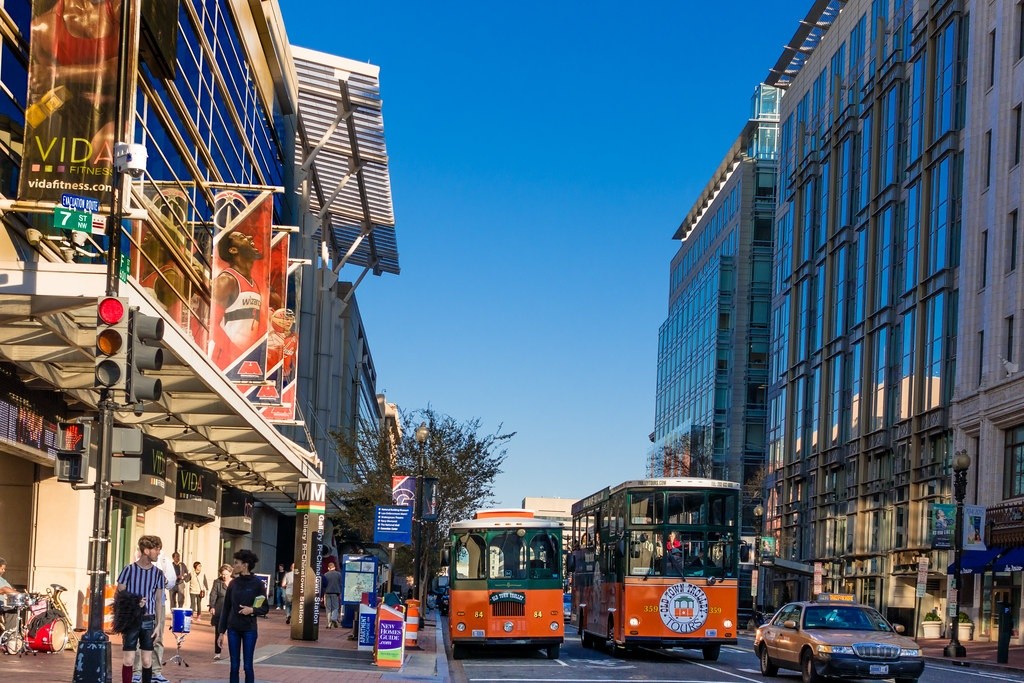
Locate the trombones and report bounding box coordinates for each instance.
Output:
[45,583,79,654]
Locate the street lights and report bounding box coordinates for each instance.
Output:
[411,422,431,630]
[753,500,764,622]
[941,449,972,659]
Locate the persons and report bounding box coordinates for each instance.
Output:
[169,552,188,609]
[667,531,681,551]
[274,565,289,611]
[141,231,181,326]
[218,550,270,683]
[688,549,716,570]
[117,535,164,683]
[763,541,770,556]
[282,563,294,624]
[935,509,954,542]
[324,562,341,629]
[282,309,297,375]
[185,562,208,621]
[213,231,263,352]
[133,552,177,683]
[29,0,122,177]
[0,557,20,612]
[209,564,236,660]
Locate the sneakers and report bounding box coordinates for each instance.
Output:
[132,674,142,682]
[151,675,169,683]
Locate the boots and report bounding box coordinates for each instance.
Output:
[197,615,201,621]
[191,615,193,621]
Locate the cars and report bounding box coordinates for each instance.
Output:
[752,589,927,683]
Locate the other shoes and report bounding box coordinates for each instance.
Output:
[213,655,221,660]
[283,605,286,609]
[286,616,291,624]
[334,620,339,628]
[276,607,281,610]
[326,622,332,628]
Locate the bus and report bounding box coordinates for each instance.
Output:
[566,474,751,664]
[437,505,578,661]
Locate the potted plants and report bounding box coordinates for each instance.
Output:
[949,611,973,641]
[921,613,942,639]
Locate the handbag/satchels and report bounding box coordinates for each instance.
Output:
[200,591,204,598]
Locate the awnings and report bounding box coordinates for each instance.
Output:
[947,546,1007,575]
[992,546,1024,572]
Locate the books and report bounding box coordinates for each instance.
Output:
[251,595,265,616]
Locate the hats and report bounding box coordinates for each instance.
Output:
[672,548,682,554]
[233,549,258,563]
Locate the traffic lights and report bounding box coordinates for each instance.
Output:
[128,311,165,406]
[93,295,130,390]
[53,420,92,484]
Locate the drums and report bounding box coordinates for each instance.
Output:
[1,629,23,655]
[24,615,68,653]
[6,592,39,607]
